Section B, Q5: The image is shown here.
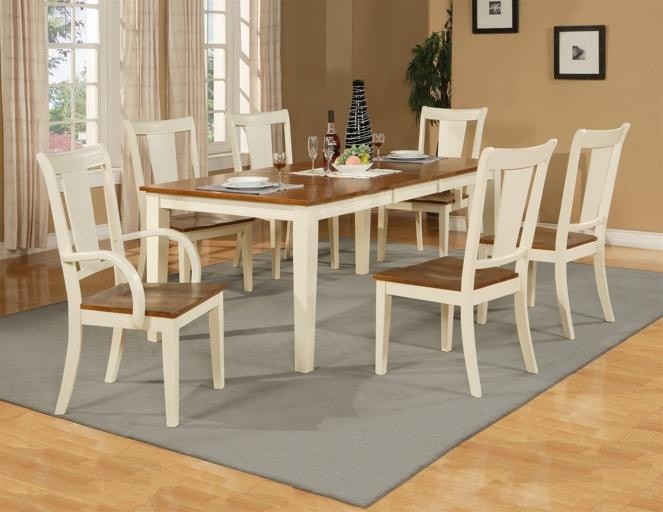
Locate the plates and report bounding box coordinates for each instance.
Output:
[225,176,269,185]
[222,183,278,190]
[386,155,429,161]
[389,149,423,156]
[331,162,374,173]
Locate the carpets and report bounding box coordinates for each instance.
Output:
[0,236,663,509]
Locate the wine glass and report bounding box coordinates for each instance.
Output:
[272,152,288,192]
[307,135,321,173]
[322,136,335,176]
[373,133,385,160]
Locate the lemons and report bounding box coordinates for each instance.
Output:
[360,157,369,164]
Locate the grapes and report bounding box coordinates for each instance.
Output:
[335,143,372,167]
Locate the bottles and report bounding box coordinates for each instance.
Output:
[323,110,341,172]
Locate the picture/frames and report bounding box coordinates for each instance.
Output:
[471,0,519,34]
[554,25,605,80]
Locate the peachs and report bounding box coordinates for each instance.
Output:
[346,156,360,165]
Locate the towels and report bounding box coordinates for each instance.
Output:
[372,154,449,164]
[288,165,402,179]
[194,181,305,194]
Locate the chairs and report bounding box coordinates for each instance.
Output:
[376,105,488,263]
[476,122,631,341]
[226,108,340,280]
[35,142,225,427]
[125,116,256,293]
[372,137,559,398]
